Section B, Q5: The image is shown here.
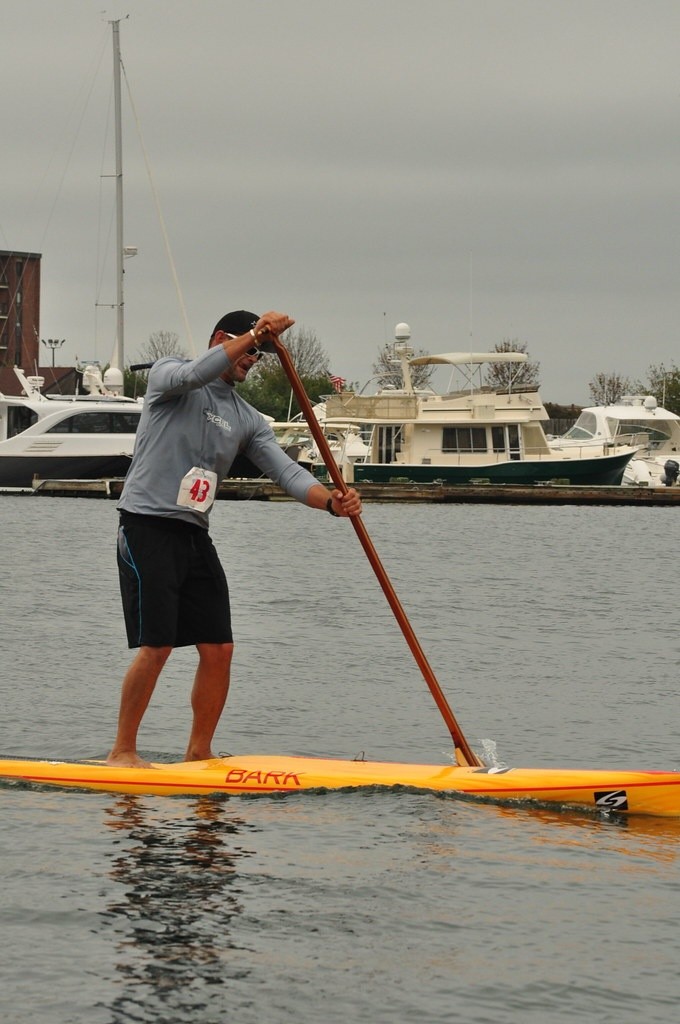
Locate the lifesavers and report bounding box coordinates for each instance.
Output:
[389,475,410,482]
[470,476,490,484]
[639,479,647,485]
[551,477,571,485]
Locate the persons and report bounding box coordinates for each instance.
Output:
[106,309,361,769]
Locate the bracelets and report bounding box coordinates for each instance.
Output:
[327,498,339,517]
[250,329,261,346]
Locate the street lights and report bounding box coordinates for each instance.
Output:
[42,339,65,367]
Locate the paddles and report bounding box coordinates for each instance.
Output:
[275,336,483,771]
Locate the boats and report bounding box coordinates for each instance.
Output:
[0,361,147,448]
[267,257,653,485]
[0,403,309,498]
[541,395,680,488]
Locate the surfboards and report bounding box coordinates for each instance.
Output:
[1,750,680,821]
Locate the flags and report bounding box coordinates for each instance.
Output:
[325,370,344,392]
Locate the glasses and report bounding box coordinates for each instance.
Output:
[225,332,264,362]
